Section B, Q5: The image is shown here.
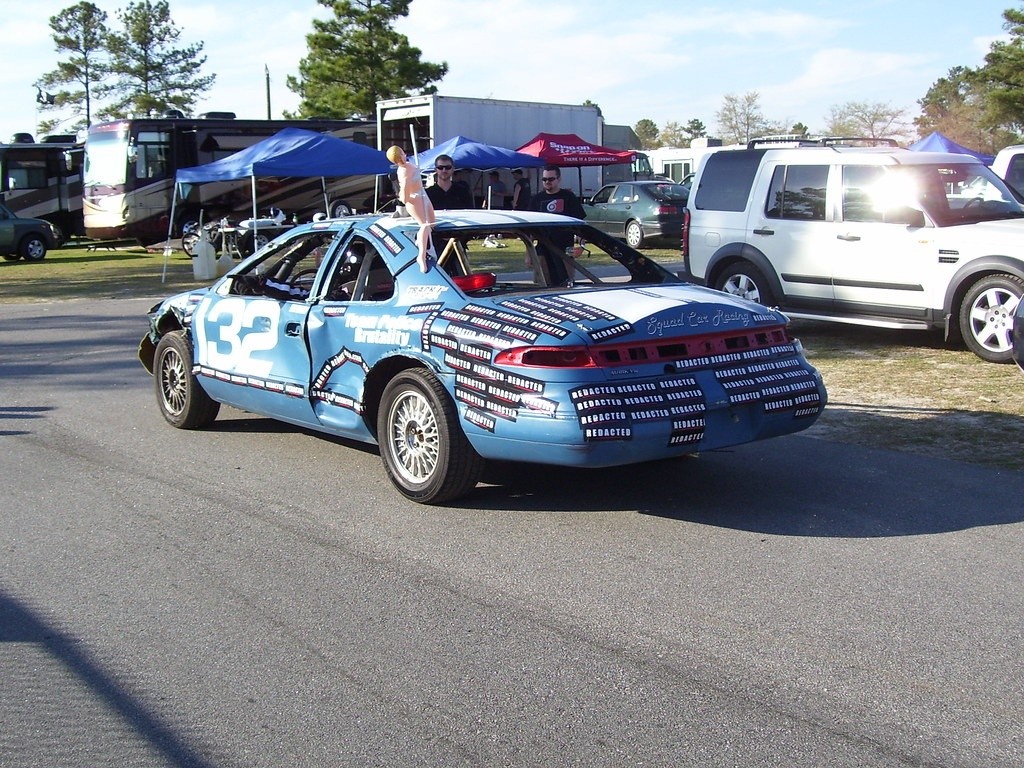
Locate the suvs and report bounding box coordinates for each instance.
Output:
[678,172,697,190]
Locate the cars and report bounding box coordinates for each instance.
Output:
[632,172,675,184]
[139,210,830,504]
[582,181,690,249]
[0,204,66,262]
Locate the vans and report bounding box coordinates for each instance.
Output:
[684,135,1024,362]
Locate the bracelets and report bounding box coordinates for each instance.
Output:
[580,244,585,248]
[512,201,517,202]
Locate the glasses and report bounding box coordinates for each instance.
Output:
[542,177,558,182]
[436,164,452,170]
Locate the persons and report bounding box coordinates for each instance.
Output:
[386,146,436,273]
[510,169,531,211]
[482,172,507,238]
[454,171,471,193]
[425,155,471,267]
[525,165,586,284]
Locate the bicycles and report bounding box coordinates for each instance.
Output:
[181,216,241,258]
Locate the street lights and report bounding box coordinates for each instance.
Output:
[264,65,273,119]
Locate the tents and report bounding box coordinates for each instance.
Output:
[161,127,638,283]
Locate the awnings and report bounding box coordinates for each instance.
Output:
[908,131,997,166]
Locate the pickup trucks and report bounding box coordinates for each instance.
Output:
[944,143,1024,207]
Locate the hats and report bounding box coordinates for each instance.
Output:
[489,171,499,176]
[511,169,523,174]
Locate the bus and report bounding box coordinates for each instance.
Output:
[77,111,381,247]
[0,134,87,244]
